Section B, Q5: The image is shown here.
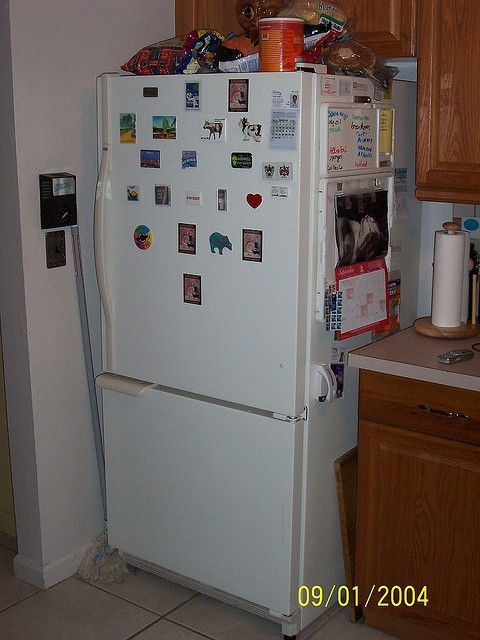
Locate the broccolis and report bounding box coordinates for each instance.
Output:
[90,70,421,640]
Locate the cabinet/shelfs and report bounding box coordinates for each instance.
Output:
[258,17,305,72]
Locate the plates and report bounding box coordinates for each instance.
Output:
[175,0,417,60]
[286,20,291,22]
[413,1,480,207]
[347,352,480,635]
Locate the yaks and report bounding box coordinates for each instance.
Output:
[69,225,152,585]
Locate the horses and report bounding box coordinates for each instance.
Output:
[438,349,475,364]
